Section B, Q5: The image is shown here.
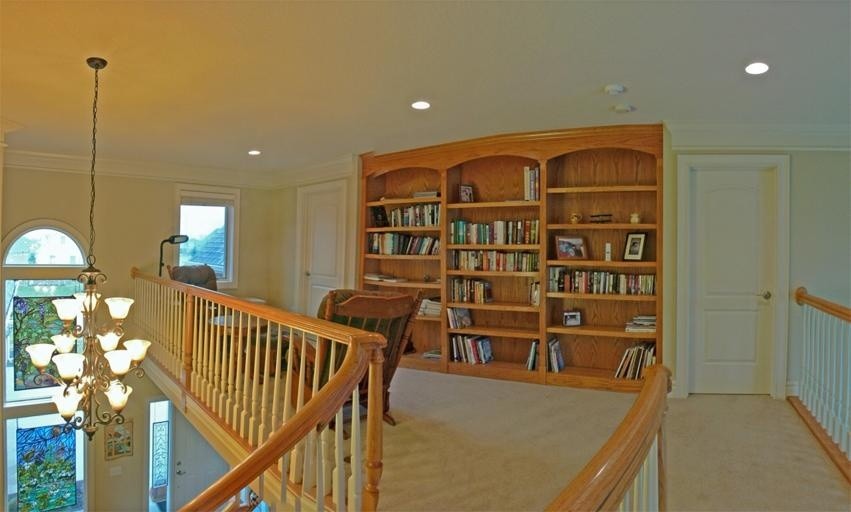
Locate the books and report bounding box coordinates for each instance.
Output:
[453,334,494,365]
[614,341,655,380]
[449,277,493,305]
[368,232,440,256]
[368,203,440,227]
[548,266,653,294]
[524,340,539,371]
[446,220,540,272]
[447,307,473,329]
[368,271,408,282]
[414,192,441,197]
[546,335,566,374]
[527,278,540,307]
[523,166,539,201]
[417,296,442,316]
[421,349,441,361]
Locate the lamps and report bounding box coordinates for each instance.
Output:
[166,264,232,336]
[281,284,427,439]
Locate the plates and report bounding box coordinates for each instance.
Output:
[355,121,677,394]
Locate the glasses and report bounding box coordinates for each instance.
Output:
[26,55,157,444]
